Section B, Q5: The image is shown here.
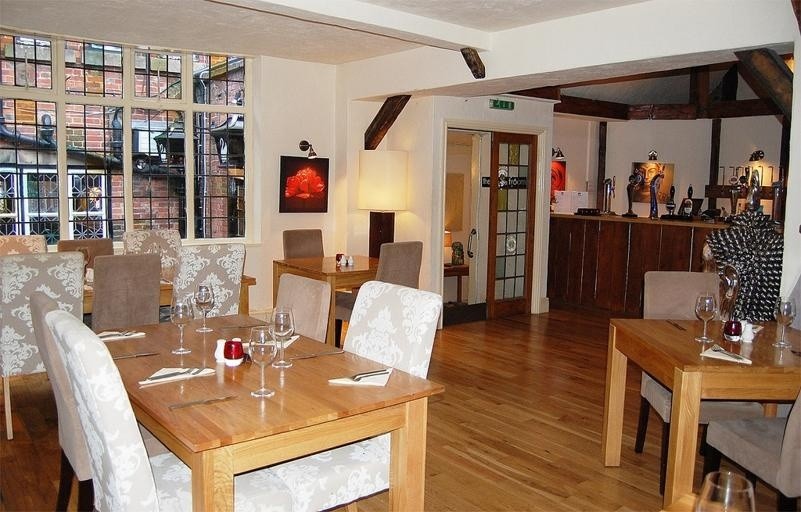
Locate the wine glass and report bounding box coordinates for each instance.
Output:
[269,306,296,368]
[249,324,277,399]
[194,283,216,334]
[695,292,717,344]
[170,292,194,356]
[772,297,797,349]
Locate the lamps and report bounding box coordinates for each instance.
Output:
[353,146,411,214]
[300,140,317,160]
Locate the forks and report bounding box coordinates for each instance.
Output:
[337,369,389,383]
[94,327,139,338]
[145,364,208,380]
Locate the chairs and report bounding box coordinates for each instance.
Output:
[641,268,801,512]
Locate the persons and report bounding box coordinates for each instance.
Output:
[631,162,671,204]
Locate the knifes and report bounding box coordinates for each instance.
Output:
[287,349,345,360]
[112,348,162,361]
[169,392,239,410]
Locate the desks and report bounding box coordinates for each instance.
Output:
[597,304,801,511]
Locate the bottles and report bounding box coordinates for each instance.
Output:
[216,337,245,366]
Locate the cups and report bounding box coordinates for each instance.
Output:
[725,321,741,346]
